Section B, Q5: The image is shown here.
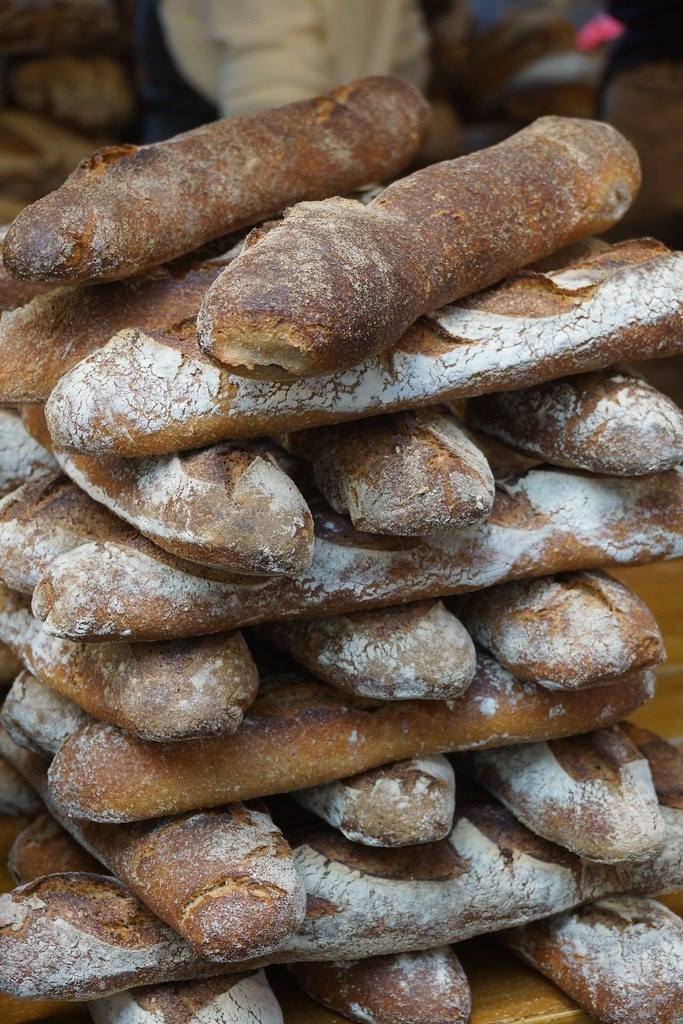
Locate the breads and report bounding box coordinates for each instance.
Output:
[0,72,683,1024]
